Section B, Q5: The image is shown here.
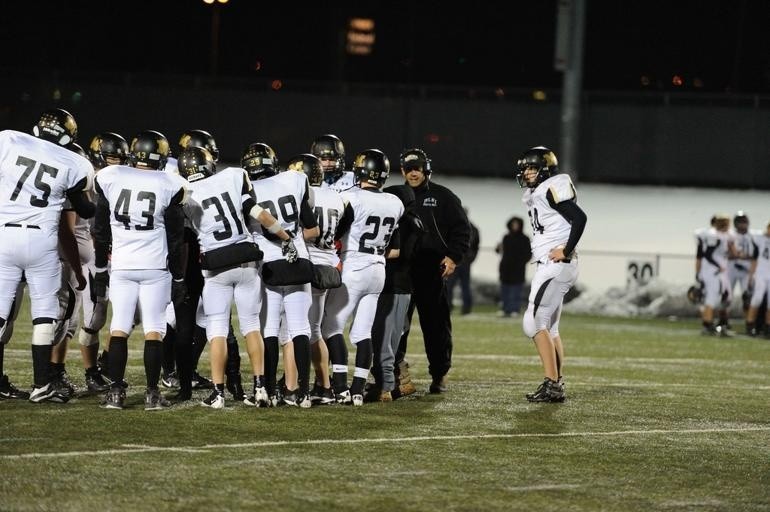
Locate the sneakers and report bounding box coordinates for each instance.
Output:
[86,372,129,393]
[430,372,447,393]
[48,370,89,398]
[144,371,416,411]
[98,385,126,409]
[30,382,69,403]
[702,317,770,339]
[526,376,565,403]
[0,376,30,400]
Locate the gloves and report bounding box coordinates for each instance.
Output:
[282,237,298,263]
[172,279,188,307]
[94,271,109,297]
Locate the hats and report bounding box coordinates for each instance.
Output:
[400,149,426,169]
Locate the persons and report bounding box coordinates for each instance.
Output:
[695,212,770,339]
[494,217,532,319]
[515,145,587,403]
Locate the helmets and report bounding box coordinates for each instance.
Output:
[178,130,219,163]
[309,134,346,177]
[89,132,129,168]
[178,146,217,182]
[687,286,703,304]
[733,212,749,230]
[515,146,558,189]
[131,129,171,171]
[711,212,730,232]
[352,149,390,188]
[33,108,89,161]
[285,153,325,186]
[239,143,279,178]
